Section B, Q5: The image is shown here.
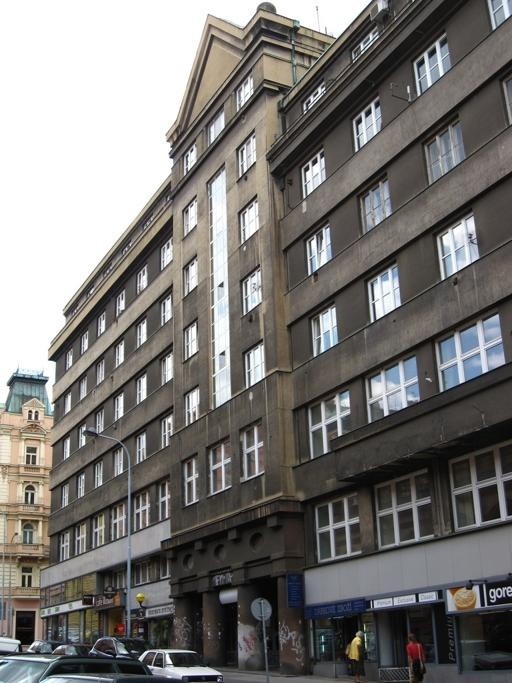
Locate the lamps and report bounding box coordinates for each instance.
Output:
[464,575,488,590]
[135,593,147,609]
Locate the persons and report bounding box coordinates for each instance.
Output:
[347,630,364,683]
[406,633,425,683]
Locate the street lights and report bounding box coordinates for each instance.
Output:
[81,425,132,640]
[7,530,20,639]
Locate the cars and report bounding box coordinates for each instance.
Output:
[461,637,512,670]
[360,630,376,661]
[0,636,223,682]
